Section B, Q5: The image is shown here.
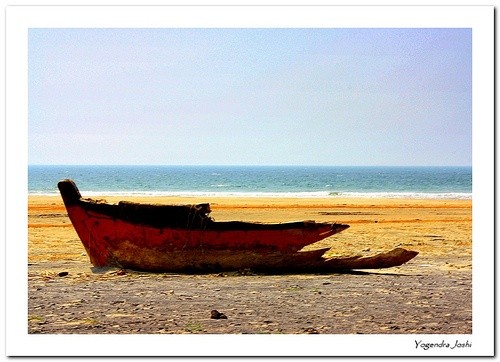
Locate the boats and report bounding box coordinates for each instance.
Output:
[56,178,420,274]
